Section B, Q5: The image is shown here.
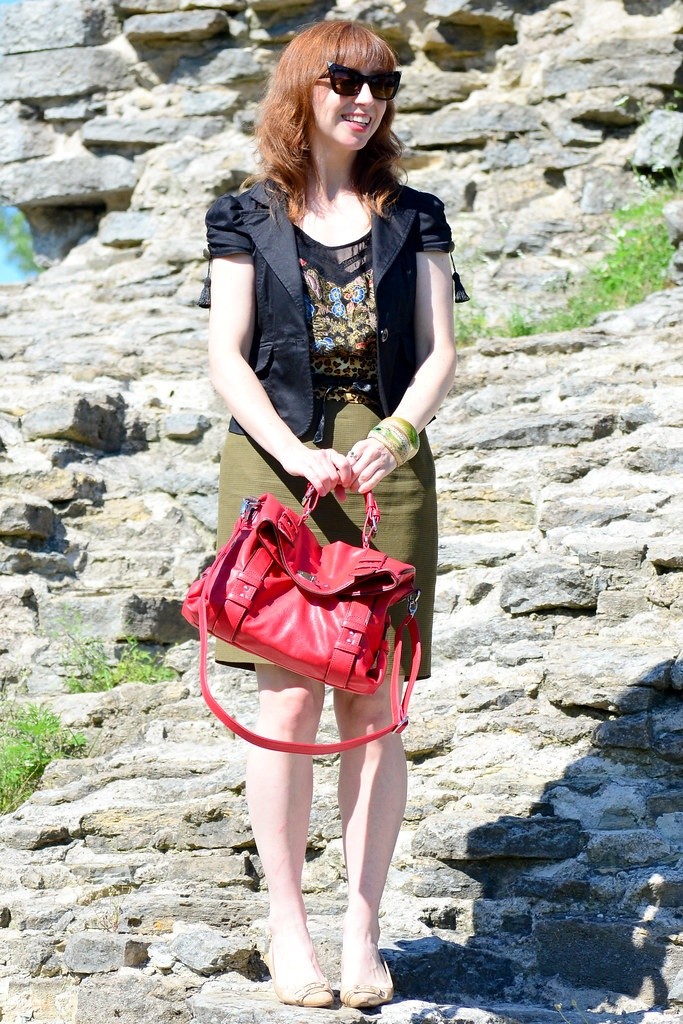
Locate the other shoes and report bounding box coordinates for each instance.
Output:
[340,950,394,1008]
[265,941,335,1007]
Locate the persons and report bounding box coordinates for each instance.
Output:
[204,18,470,1009]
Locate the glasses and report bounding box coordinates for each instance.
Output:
[318,61,402,100]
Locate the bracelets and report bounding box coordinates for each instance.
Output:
[368,416,420,468]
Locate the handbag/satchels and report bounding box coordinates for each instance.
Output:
[181,473,422,755]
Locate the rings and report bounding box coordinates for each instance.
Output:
[348,451,359,461]
